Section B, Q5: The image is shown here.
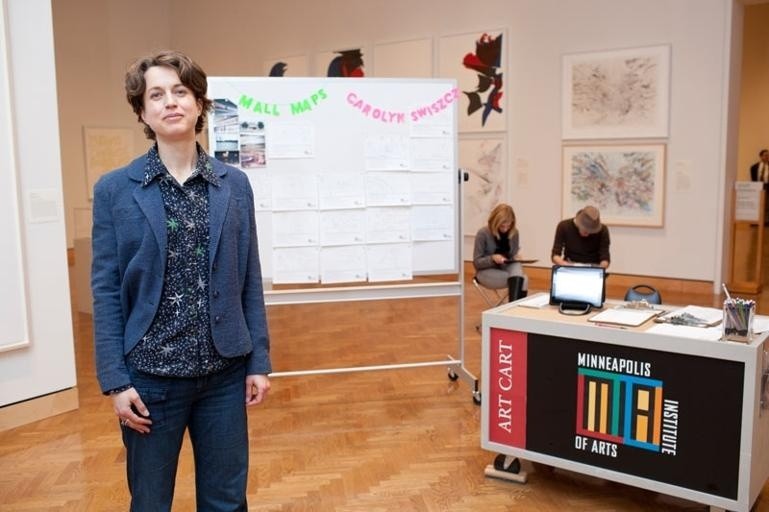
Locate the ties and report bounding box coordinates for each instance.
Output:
[760,162,766,181]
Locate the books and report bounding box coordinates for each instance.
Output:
[588,300,665,330]
[656,303,724,327]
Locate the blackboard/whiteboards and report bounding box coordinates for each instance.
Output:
[205,75,467,302]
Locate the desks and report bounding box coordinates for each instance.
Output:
[480,292,769,512]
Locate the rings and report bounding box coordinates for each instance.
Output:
[123,419,131,425]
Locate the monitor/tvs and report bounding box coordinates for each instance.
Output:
[550,265,605,309]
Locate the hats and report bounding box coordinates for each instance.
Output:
[575,206,602,234]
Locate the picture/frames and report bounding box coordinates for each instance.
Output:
[74,208,93,239]
[560,141,667,229]
[82,125,135,201]
[456,133,510,238]
[371,37,434,78]
[436,25,510,133]
[312,47,369,77]
[560,43,672,140]
[1,2,32,354]
[260,55,311,78]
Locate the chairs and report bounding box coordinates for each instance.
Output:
[472,277,511,331]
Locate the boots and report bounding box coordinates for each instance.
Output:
[508,277,524,301]
[521,290,527,297]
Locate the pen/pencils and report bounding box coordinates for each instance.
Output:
[595,322,628,330]
[658,310,666,318]
[721,282,731,300]
[568,258,575,266]
[723,297,755,343]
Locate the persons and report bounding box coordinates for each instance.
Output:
[474,206,527,302]
[750,150,769,188]
[553,207,610,270]
[93,49,272,509]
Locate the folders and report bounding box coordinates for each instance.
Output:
[589,299,670,332]
[655,306,723,330]
